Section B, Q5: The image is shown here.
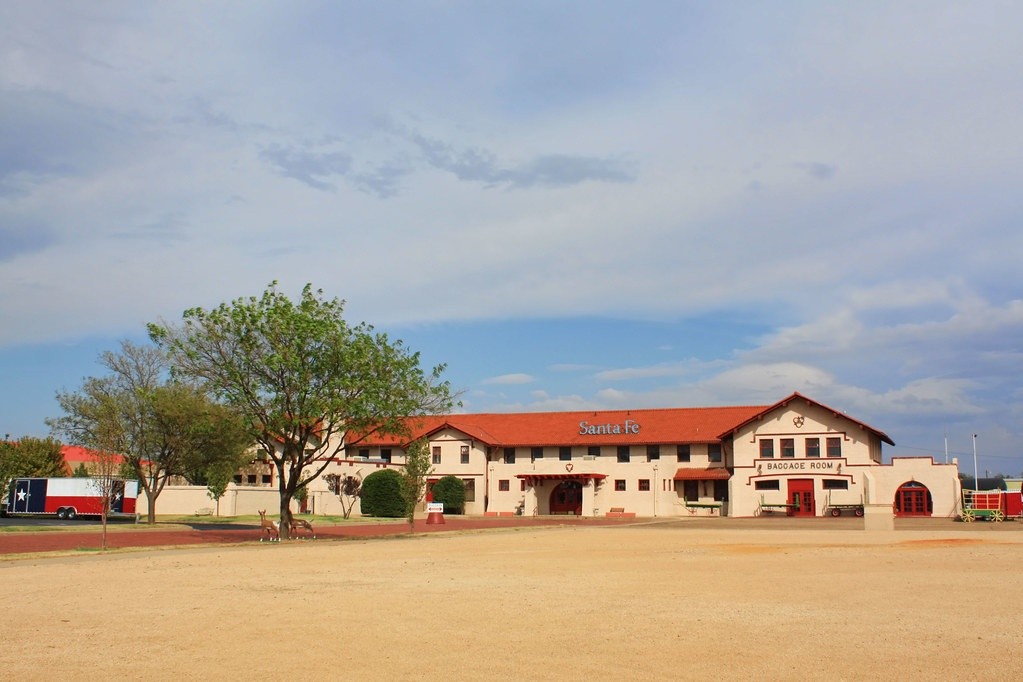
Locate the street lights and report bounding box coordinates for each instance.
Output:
[972,433,977,490]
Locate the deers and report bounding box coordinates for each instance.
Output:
[259,509,281,542]
[288,509,316,540]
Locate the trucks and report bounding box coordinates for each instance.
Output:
[2,478,138,522]
